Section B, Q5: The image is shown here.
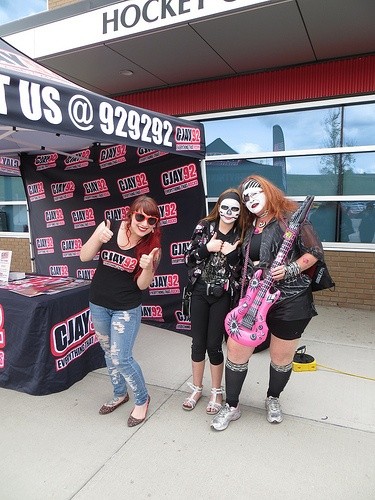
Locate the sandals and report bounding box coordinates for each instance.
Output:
[206,386,223,415]
[183,382,203,410]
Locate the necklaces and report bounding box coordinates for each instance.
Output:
[257,210,271,228]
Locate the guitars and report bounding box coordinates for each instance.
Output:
[224,195,315,346]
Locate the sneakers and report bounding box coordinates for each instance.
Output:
[265,396,283,423]
[210,402,241,430]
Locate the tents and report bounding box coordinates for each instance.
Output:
[206,138,284,196]
[1,38,210,337]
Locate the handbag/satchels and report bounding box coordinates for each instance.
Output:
[305,260,335,293]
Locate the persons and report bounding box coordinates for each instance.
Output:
[210,175,323,431]
[80,196,162,427]
[310,201,375,243]
[184,188,246,415]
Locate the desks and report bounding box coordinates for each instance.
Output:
[0,275,107,396]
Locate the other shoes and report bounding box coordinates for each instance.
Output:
[99,392,129,415]
[127,395,150,426]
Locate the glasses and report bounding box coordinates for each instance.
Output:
[133,212,158,226]
